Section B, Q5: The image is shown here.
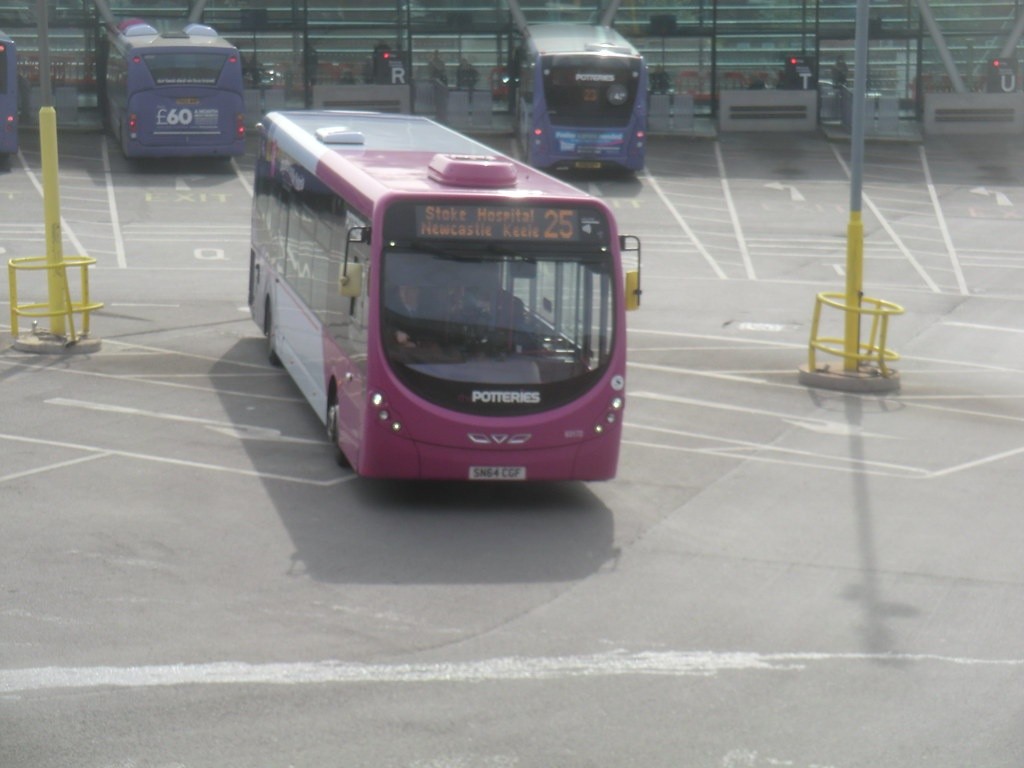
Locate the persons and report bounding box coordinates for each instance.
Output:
[748,57,852,95]
[244,39,480,92]
[648,64,672,96]
[379,272,437,356]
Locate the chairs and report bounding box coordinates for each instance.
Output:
[502,290,525,325]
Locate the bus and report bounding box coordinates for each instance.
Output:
[512,20,650,178]
[101,15,248,160]
[247,107,643,495]
[0,32,22,155]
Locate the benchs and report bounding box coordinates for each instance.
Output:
[906,74,969,102]
[277,62,352,92]
[676,72,775,102]
[16,54,81,86]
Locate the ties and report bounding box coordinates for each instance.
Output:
[410,309,414,316]
